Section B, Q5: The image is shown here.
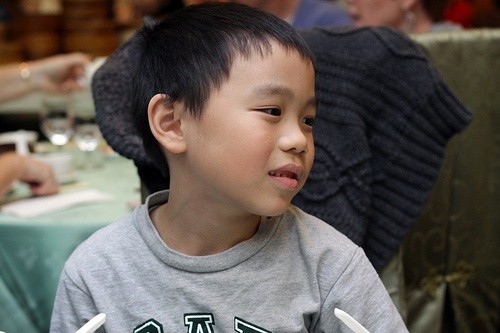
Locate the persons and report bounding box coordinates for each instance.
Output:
[0,50,90,105]
[49,2,410,333]
[345,0,463,34]
[0,152,58,199]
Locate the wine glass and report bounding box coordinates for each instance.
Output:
[41,90,102,172]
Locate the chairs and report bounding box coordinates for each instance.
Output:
[91,22,473,321]
[404,28,500,333]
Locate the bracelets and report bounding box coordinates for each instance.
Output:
[21,61,34,91]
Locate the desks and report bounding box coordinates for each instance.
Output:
[0,144,141,333]
